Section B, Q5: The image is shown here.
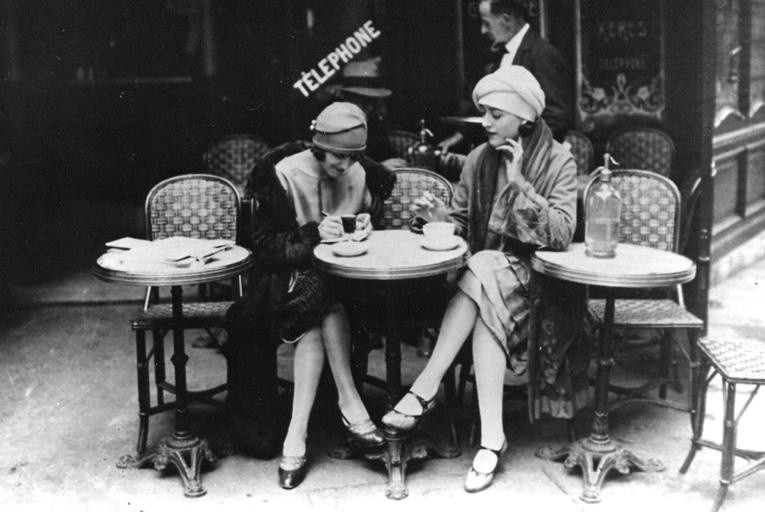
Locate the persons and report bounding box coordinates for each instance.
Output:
[379,66,577,493]
[226,103,396,488]
[438,0,575,159]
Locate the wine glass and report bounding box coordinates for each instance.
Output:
[338,212,357,247]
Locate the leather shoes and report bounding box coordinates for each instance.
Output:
[336,391,436,447]
[465,440,508,492]
[279,456,308,488]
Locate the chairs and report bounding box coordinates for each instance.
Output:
[564,128,593,178]
[675,164,703,255]
[678,336,765,512]
[391,130,419,157]
[377,168,452,231]
[574,168,703,414]
[130,174,243,454]
[606,125,677,179]
[204,135,269,203]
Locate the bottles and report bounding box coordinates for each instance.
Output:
[582,169,626,259]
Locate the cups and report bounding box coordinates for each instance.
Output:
[423,220,454,245]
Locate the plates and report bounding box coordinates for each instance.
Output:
[328,241,368,257]
[420,241,460,253]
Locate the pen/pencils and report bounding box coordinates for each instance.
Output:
[321,211,328,216]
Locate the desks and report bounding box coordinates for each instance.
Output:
[530,241,697,502]
[96,237,253,496]
[314,228,470,501]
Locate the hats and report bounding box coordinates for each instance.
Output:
[472,65,546,123]
[310,102,368,153]
[342,62,392,98]
